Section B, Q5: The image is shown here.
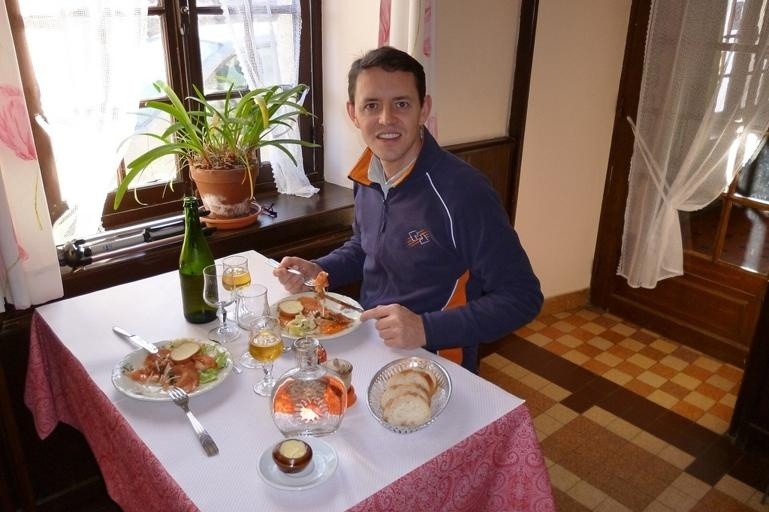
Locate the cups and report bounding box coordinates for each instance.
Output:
[320,358,353,393]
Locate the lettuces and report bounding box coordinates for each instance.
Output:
[196,342,229,384]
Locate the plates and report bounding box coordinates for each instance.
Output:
[256,437,338,491]
[270,291,365,340]
[111,338,234,403]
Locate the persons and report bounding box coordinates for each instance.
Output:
[273,47,543,378]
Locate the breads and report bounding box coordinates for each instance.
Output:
[379,367,438,427]
[278,300,304,316]
[169,341,202,365]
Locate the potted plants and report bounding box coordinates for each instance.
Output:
[115,73,323,218]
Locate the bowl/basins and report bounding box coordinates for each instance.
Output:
[366,356,453,434]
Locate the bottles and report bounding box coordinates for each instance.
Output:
[177,196,220,325]
[269,335,348,438]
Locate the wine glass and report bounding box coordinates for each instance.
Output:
[221,254,252,322]
[202,263,240,342]
[237,282,271,369]
[249,314,285,397]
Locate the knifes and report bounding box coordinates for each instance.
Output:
[324,293,382,321]
[112,326,159,354]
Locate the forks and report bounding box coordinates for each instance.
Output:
[167,386,221,458]
[264,259,327,289]
